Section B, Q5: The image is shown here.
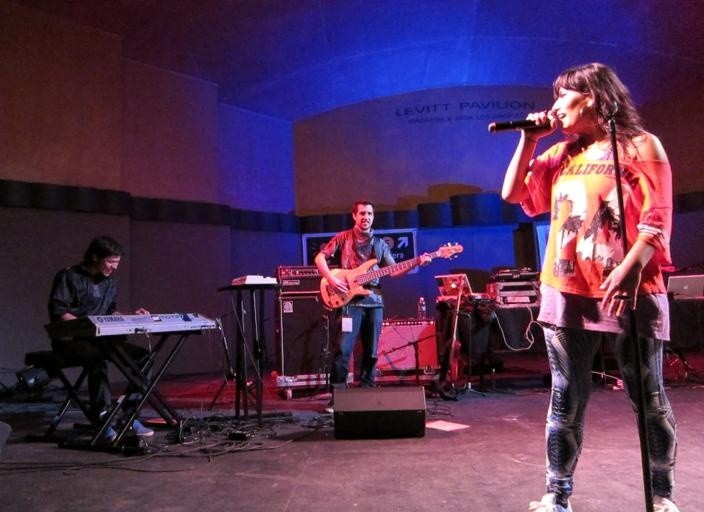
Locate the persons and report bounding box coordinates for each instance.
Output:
[313,199,431,413]
[45,235,155,440]
[499,61,682,512]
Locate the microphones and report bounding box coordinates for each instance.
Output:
[488,113,559,134]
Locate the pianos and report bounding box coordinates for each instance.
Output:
[45,312,218,340]
[232,275,277,286]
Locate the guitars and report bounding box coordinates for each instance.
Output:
[321,241,464,308]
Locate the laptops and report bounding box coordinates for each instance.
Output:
[667,274,704,300]
[434,273,484,295]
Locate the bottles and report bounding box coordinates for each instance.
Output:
[416,296,427,321]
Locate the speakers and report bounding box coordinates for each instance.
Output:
[274,296,334,379]
[332,385,427,441]
[353,319,442,372]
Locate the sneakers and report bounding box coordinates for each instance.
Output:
[532,492,572,512]
[324,397,334,413]
[101,420,153,444]
[653,494,679,512]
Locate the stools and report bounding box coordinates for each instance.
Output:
[26,343,150,443]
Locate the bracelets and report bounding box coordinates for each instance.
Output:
[519,134,538,143]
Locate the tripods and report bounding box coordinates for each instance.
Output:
[457,317,487,397]
